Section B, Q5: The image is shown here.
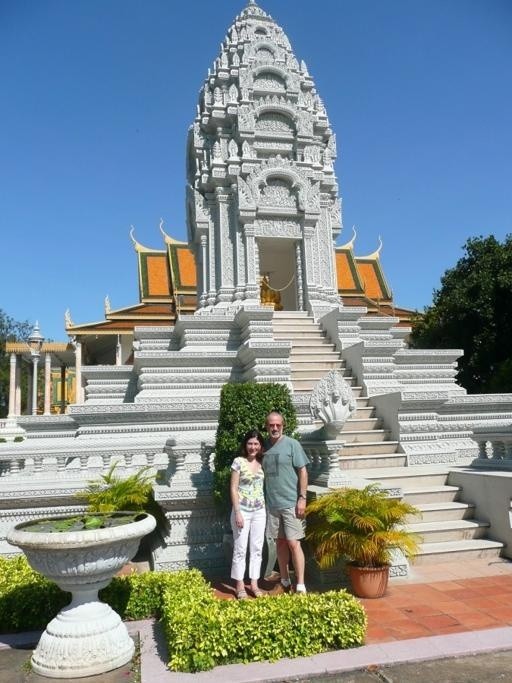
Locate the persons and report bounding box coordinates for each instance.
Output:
[257,409,313,594]
[227,430,269,598]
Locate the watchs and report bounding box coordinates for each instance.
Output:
[298,494,307,499]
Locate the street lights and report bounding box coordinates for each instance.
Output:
[25,316,46,415]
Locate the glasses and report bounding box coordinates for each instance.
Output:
[269,423,281,427]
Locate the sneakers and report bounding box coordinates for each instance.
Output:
[267,583,294,595]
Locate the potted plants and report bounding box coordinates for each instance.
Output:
[295,482,424,598]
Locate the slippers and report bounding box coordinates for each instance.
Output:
[251,587,263,597]
[235,587,247,600]
